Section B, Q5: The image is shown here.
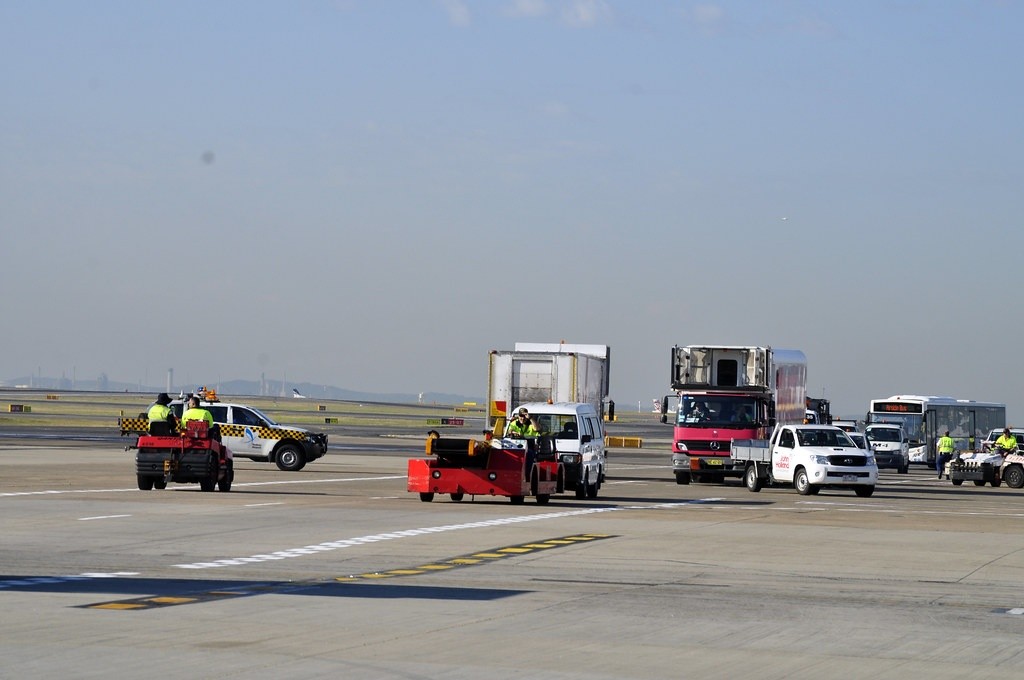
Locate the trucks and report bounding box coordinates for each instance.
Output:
[658,342,832,485]
[485,342,615,441]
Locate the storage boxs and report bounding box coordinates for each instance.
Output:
[186,420,209,438]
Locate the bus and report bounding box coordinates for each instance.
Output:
[864,393,1008,469]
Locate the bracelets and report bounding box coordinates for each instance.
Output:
[529,416,532,421]
[511,430,514,434]
[998,445,1001,448]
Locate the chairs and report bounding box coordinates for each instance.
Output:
[150,422,171,436]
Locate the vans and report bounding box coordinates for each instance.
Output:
[727,417,1024,498]
[492,400,609,499]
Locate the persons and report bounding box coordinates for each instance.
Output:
[995,428,1017,458]
[148,393,178,434]
[508,408,540,482]
[937,430,957,480]
[181,397,214,438]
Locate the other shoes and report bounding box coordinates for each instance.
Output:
[939,471,942,479]
[946,475,950,480]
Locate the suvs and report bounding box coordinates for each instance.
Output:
[144,387,329,471]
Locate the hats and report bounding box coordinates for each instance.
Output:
[155,393,173,404]
[695,403,702,406]
[519,408,528,414]
